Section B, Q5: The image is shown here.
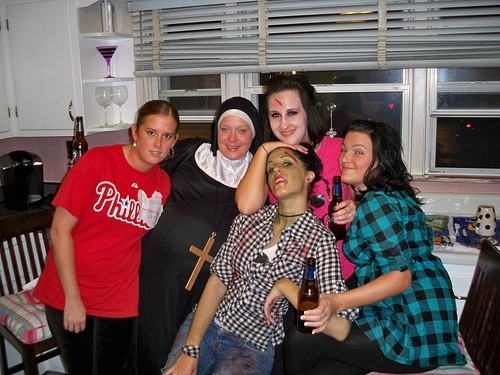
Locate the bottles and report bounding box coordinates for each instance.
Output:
[328,176,346,240]
[296,257,319,333]
[72,116,88,164]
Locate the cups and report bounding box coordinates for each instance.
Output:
[475,206,497,236]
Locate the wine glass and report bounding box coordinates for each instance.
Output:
[96,46,119,78]
[95,86,114,128]
[111,86,130,126]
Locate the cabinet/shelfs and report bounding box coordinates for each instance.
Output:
[0,0,138,141]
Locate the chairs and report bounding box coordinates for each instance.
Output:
[0,211,62,375]
[368,239,500,375]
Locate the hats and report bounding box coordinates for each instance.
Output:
[209,97,265,157]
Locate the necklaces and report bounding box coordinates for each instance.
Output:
[277,212,304,218]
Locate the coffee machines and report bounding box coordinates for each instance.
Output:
[0,150,45,210]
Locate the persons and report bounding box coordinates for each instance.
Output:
[235,76,357,375]
[33,100,180,375]
[301,121,468,375]
[161,143,359,375]
[138,97,263,375]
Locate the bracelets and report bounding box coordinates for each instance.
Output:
[180,345,201,358]
[260,144,268,155]
